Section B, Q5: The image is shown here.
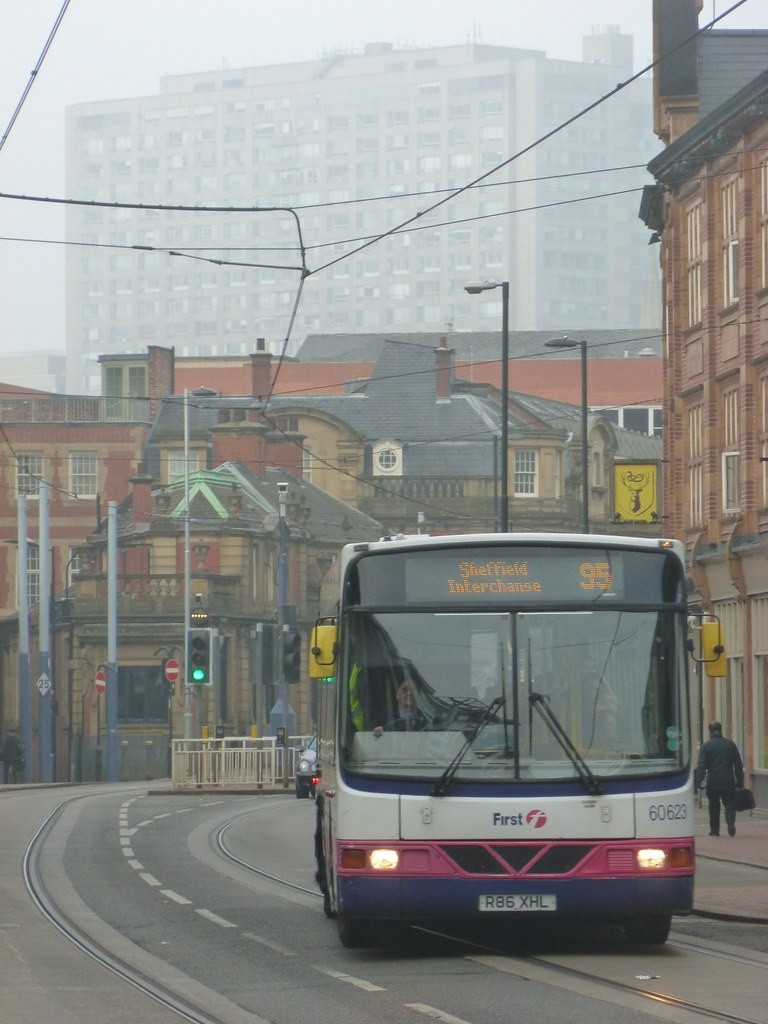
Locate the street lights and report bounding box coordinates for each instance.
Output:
[5,538,56,782]
[544,335,588,532]
[182,386,217,739]
[493,432,525,533]
[463,279,509,532]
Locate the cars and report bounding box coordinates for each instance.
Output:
[295,734,317,799]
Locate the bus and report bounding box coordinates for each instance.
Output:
[309,532,728,947]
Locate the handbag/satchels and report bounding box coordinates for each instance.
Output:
[731,787,755,812]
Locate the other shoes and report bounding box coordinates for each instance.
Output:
[708,832,719,836]
[726,816,736,836]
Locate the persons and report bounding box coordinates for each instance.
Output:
[694,721,744,838]
[374,640,620,752]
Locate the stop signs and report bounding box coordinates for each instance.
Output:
[164,659,180,683]
[95,671,106,695]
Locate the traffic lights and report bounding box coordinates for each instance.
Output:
[279,622,303,685]
[185,628,213,687]
